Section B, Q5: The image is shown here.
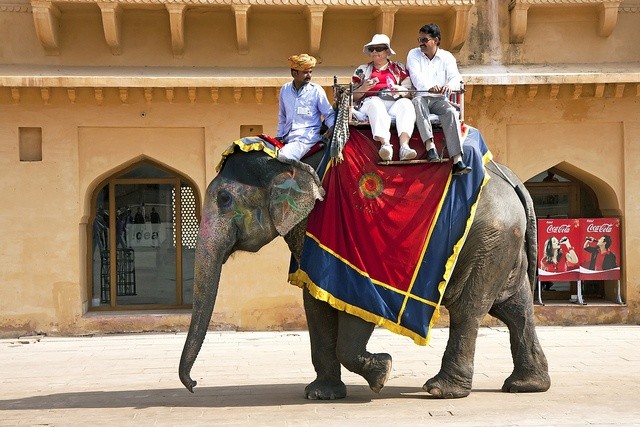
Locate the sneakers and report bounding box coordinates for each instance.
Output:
[399,145,417,160]
[378,143,393,161]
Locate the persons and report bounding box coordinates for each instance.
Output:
[275,52,338,163]
[406,23,471,176]
[582,233,617,269]
[351,32,417,161]
[540,235,579,272]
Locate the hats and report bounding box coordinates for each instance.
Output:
[363,34,397,57]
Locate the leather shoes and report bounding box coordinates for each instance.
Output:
[427,148,439,162]
[452,162,473,175]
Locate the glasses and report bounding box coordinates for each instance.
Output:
[417,37,434,44]
[368,46,388,52]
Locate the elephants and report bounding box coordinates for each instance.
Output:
[179,134,551,401]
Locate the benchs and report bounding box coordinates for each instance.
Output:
[332,75,466,131]
